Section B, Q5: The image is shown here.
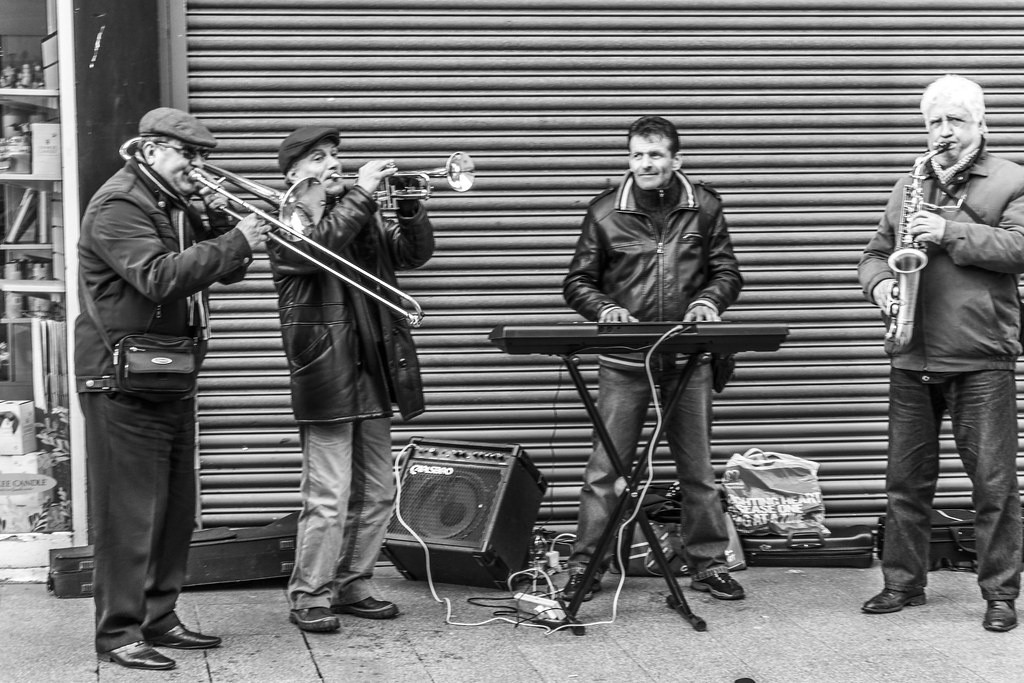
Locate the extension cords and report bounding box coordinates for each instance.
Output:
[514,592,567,620]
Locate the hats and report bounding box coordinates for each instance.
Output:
[279,125,341,174]
[139,107,218,150]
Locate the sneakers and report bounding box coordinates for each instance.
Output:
[330,597,398,619]
[288,605,341,633]
[561,575,601,601]
[691,573,743,600]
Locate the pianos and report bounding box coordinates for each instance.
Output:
[487,324,789,636]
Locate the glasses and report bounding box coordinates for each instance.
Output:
[156,141,210,159]
[915,184,970,212]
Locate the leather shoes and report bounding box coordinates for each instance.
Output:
[98,638,176,669]
[146,626,221,648]
[861,587,927,612]
[983,597,1018,630]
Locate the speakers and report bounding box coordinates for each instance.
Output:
[381,436,548,590]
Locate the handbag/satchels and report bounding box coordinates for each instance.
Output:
[721,447,826,531]
[613,483,744,571]
[112,336,197,396]
[707,352,735,392]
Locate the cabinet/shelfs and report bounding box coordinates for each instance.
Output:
[0,88,66,294]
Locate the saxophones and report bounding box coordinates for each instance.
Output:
[884,143,952,356]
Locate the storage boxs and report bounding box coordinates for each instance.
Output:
[0,400,52,476]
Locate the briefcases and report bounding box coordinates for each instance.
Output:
[876,508,981,572]
[738,524,874,567]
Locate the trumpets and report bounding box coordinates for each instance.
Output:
[330,151,475,210]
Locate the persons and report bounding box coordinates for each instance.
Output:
[264,127,435,631]
[856,73,1024,631]
[74,107,271,669]
[560,116,744,599]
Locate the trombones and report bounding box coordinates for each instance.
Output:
[119,136,426,324]
